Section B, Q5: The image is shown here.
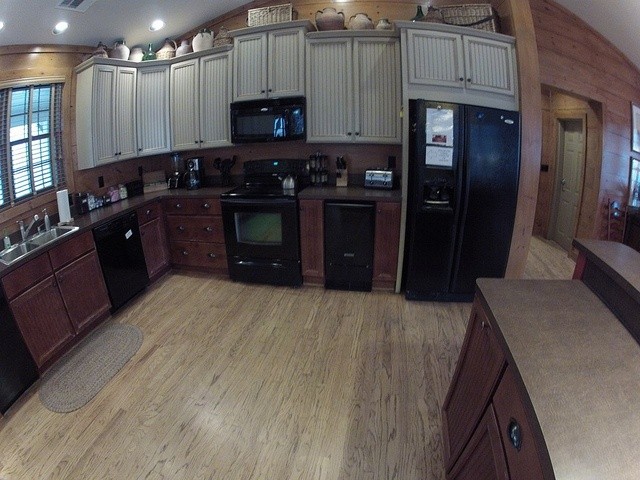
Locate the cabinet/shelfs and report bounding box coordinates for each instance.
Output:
[306,29,402,146]
[571,236,639,342]
[439,278,508,473]
[372,200,402,291]
[137,58,170,157]
[226,19,306,103]
[134,197,173,284]
[168,43,233,152]
[396,21,522,112]
[1,230,113,378]
[299,197,326,287]
[164,195,229,275]
[448,365,556,480]
[72,57,137,171]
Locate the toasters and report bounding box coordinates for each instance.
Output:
[363,167,394,190]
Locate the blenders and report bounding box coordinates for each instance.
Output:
[167,153,185,189]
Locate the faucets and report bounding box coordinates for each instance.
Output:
[17,214,40,241]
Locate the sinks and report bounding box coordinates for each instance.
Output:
[30,228,72,244]
[1,244,38,263]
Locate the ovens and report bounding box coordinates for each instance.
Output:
[221,199,303,287]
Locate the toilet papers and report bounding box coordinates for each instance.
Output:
[56,189,71,223]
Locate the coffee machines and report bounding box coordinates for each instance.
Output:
[183,156,204,189]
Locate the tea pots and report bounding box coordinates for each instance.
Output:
[278,173,299,191]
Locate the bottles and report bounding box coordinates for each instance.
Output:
[119,183,128,200]
[109,185,119,203]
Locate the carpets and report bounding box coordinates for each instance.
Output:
[37,321,143,412]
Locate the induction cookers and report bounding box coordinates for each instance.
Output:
[221,180,311,199]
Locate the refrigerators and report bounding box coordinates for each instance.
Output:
[404,98,520,303]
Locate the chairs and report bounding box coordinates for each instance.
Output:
[608,198,628,243]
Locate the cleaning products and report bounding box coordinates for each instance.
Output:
[42,208,51,231]
[4,236,12,250]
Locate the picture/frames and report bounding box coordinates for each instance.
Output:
[630,100,640,154]
[627,156,640,208]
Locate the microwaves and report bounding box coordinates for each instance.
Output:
[230,96,305,143]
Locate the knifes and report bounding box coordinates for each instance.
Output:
[336,156,346,169]
